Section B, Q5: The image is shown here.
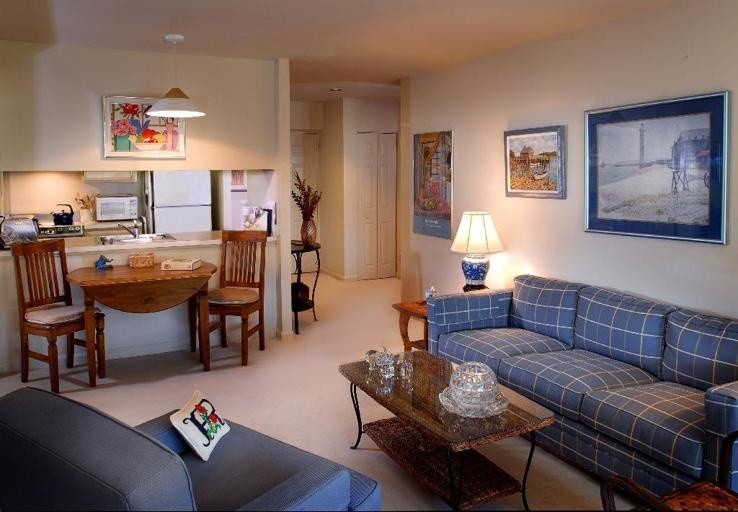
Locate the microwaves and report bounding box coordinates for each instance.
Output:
[94,195,138,222]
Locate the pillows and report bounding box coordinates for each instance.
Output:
[169,390,231,462]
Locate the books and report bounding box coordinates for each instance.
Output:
[160,257,202,271]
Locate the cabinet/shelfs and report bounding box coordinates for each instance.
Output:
[291,240,321,335]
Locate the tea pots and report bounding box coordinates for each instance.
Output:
[48,203,74,226]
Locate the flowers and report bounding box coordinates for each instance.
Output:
[291,168,323,220]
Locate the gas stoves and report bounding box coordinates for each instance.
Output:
[35,221,86,238]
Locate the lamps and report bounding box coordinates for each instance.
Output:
[449,210,505,293]
[144,35,207,118]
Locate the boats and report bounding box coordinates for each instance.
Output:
[529,161,541,165]
[533,171,549,180]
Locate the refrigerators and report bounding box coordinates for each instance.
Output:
[143,169,212,233]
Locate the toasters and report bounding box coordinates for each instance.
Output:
[0,217,40,249]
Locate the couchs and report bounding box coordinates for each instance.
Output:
[428,274,738,509]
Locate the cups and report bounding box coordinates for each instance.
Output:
[360,347,415,393]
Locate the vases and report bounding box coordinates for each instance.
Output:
[301,220,317,249]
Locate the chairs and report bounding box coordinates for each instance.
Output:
[0,386,382,511]
[196,230,269,367]
[11,238,106,392]
[601,430,738,511]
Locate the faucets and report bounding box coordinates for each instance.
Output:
[117,223,139,239]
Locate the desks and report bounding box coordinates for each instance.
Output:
[392,299,428,353]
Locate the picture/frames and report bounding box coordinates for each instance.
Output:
[101,95,186,160]
[503,124,566,199]
[584,90,731,246]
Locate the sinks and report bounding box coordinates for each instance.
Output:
[104,233,176,243]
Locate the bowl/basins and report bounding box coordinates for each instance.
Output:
[99,235,113,245]
[436,386,509,420]
[439,406,509,431]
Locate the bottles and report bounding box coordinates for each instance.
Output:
[240,200,250,230]
